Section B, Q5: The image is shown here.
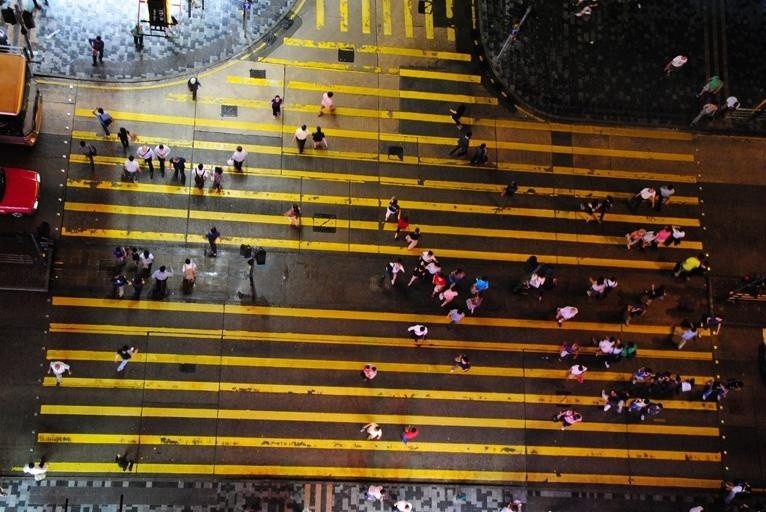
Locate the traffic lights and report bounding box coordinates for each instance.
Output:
[256,248,266,265]
[1,6,15,25]
[733,272,753,288]
[22,10,36,30]
[749,283,765,298]
[239,243,251,257]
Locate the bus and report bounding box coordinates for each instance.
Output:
[0,51,42,150]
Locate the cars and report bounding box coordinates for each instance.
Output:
[0,166,41,219]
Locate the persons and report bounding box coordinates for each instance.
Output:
[357,364,420,509]
[113,343,138,373]
[22,457,48,477]
[381,195,491,373]
[108,225,220,302]
[663,52,742,129]
[113,452,134,471]
[286,204,303,228]
[449,105,517,207]
[79,25,336,193]
[578,183,688,253]
[48,359,71,387]
[504,253,766,511]
